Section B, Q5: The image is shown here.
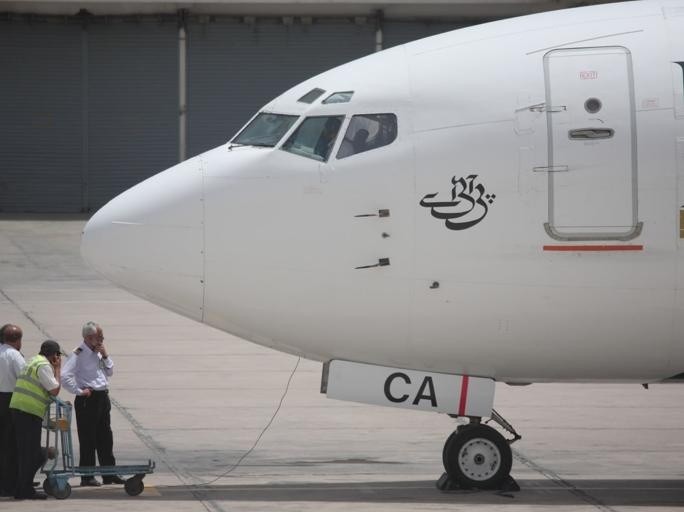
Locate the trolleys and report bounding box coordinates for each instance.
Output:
[40,395,155,499]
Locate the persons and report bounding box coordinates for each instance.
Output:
[324,118,355,159]
[61,321,128,485]
[0,324,27,495]
[7,341,60,498]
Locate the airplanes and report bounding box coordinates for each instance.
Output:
[80,0,684,491]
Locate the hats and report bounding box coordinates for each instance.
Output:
[41,340,62,356]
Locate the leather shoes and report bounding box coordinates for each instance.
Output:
[80,478,101,486]
[103,475,126,484]
[0,481,48,500]
